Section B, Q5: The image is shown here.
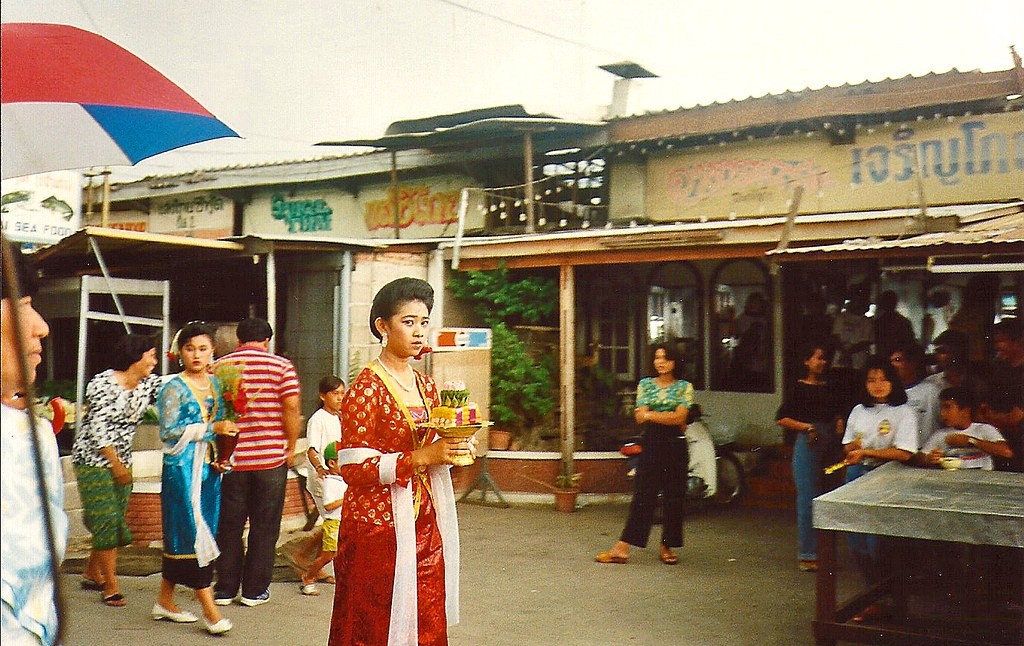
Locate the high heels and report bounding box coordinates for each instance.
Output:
[151,603,197,624]
[201,615,232,635]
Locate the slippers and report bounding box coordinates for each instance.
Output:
[661,551,678,564]
[81,581,106,591]
[101,591,126,607]
[595,552,626,563]
[300,584,316,595]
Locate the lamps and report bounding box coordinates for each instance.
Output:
[926,256,1024,273]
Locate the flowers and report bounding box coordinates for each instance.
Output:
[216,362,264,421]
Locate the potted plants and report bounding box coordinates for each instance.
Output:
[489,322,554,451]
[552,461,583,512]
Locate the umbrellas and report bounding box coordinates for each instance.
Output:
[0,22,245,180]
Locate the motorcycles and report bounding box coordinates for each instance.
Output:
[621,402,746,512]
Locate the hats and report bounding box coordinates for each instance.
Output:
[325,441,342,459]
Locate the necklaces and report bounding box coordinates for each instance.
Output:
[377,356,417,392]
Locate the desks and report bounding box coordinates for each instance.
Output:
[810,460,1024,646]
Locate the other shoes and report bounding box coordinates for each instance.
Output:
[799,560,816,572]
[215,595,238,605]
[239,590,270,607]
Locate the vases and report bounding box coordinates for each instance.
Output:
[215,434,239,464]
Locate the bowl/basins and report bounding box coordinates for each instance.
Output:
[939,457,963,471]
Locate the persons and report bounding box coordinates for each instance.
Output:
[0,238,68,646]
[212,316,302,607]
[726,289,1024,623]
[294,375,346,596]
[71,334,165,608]
[327,277,479,646]
[596,341,695,564]
[151,320,240,633]
[685,405,717,498]
